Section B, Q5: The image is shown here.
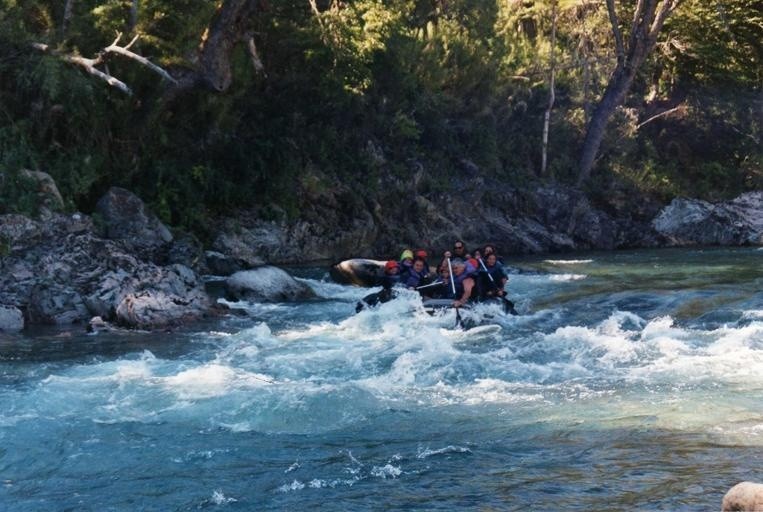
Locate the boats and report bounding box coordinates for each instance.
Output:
[354,284,511,329]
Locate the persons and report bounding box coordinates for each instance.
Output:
[381,239,509,309]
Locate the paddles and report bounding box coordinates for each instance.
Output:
[447,257,464,328]
[479,258,518,316]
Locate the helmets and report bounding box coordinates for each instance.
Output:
[384,250,427,271]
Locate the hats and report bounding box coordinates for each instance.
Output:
[468,258,479,268]
